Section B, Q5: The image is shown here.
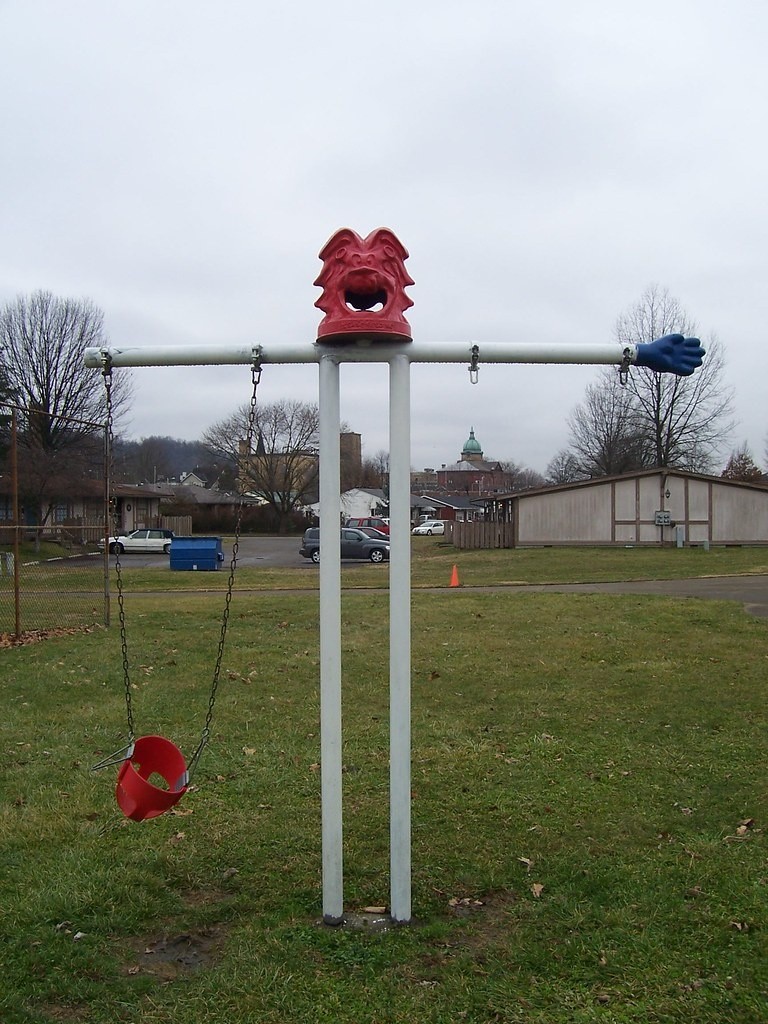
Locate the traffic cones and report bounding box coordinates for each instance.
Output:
[448,564,460,587]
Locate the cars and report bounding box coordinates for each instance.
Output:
[96,529,175,554]
[345,527,390,542]
[411,521,445,536]
[381,518,390,526]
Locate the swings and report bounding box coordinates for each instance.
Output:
[99,364,262,824]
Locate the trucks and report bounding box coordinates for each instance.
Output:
[411,515,451,526]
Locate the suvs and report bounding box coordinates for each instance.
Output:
[299,527,390,564]
[344,516,389,535]
